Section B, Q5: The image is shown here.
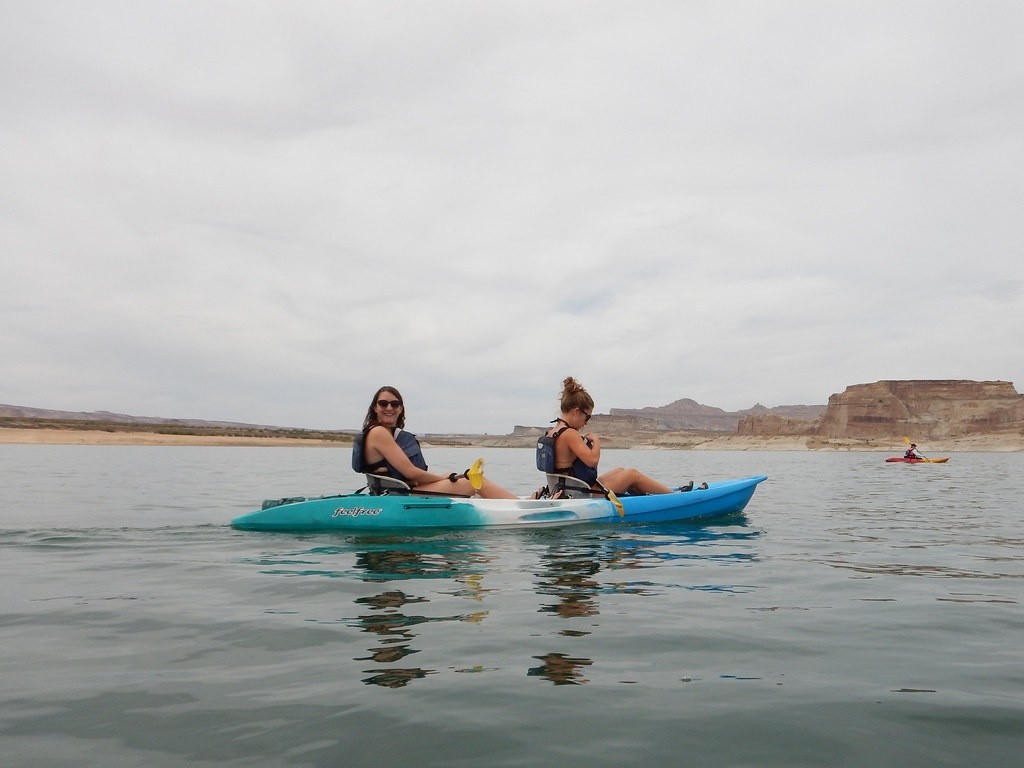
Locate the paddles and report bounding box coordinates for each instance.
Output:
[446,457,485,490]
[595,479,625,518]
[904,437,932,463]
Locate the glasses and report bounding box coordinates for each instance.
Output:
[578,407,592,422]
[377,400,401,408]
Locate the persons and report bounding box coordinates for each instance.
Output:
[546,377,709,497]
[906,444,925,459]
[362,387,565,499]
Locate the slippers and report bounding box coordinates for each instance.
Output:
[678,481,694,493]
[532,486,545,500]
[551,490,564,500]
[696,482,708,491]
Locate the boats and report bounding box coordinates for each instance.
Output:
[884,456,949,464]
[229,468,768,534]
